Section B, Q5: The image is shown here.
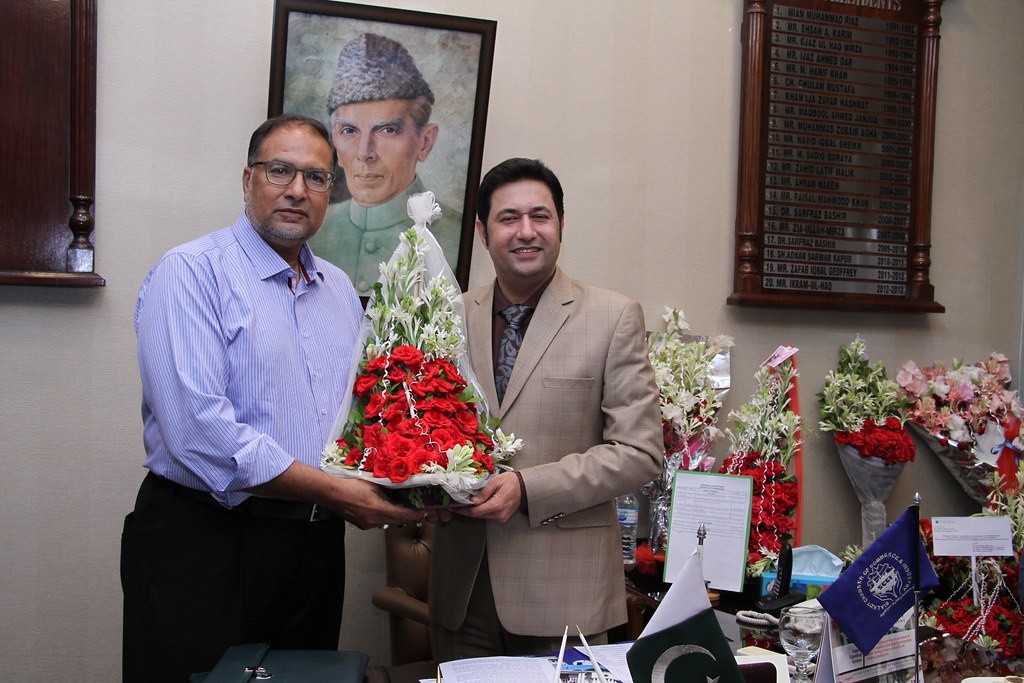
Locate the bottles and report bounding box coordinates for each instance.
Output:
[615,492,639,565]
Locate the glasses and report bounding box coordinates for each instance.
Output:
[251,160,336,194]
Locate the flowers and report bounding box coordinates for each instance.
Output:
[814,332,918,550]
[838,459,1024,668]
[718,355,822,578]
[895,350,1024,504]
[644,305,736,551]
[320,227,526,490]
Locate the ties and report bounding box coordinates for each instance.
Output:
[494,304,535,409]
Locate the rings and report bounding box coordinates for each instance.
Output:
[379,524,390,530]
[416,523,424,527]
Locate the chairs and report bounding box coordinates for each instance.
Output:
[373,523,651,683]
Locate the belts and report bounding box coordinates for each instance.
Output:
[151,473,334,522]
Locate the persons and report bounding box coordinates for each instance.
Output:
[120,113,437,683]
[431,157,667,661]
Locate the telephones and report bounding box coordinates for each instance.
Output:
[790,598,829,635]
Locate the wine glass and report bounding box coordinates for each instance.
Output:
[778,606,825,683]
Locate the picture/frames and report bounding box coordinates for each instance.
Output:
[267,0,498,314]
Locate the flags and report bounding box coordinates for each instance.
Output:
[626,544,742,683]
[816,504,940,655]
